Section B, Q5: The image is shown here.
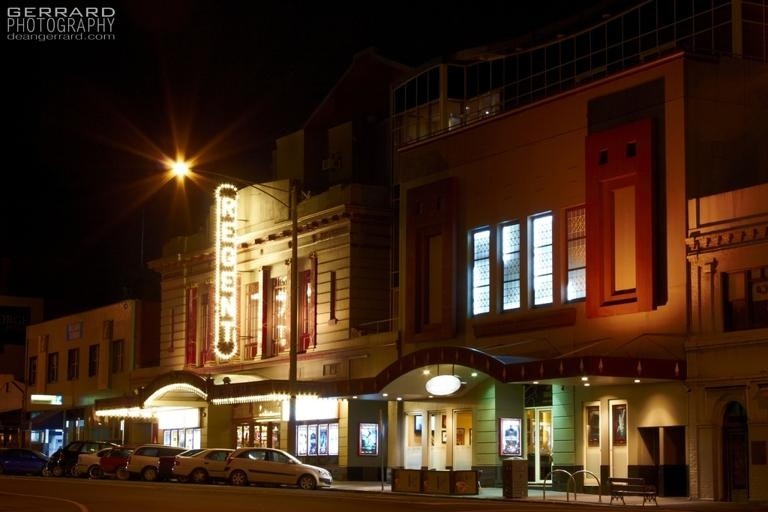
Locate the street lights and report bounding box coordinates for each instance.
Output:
[170,162,298,452]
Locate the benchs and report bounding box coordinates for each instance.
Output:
[608,476,658,506]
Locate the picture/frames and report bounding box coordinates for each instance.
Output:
[442,431,446,443]
[585,406,600,447]
[612,404,627,446]
[358,422,379,457]
[499,417,523,458]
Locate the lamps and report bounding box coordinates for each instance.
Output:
[426,365,461,396]
